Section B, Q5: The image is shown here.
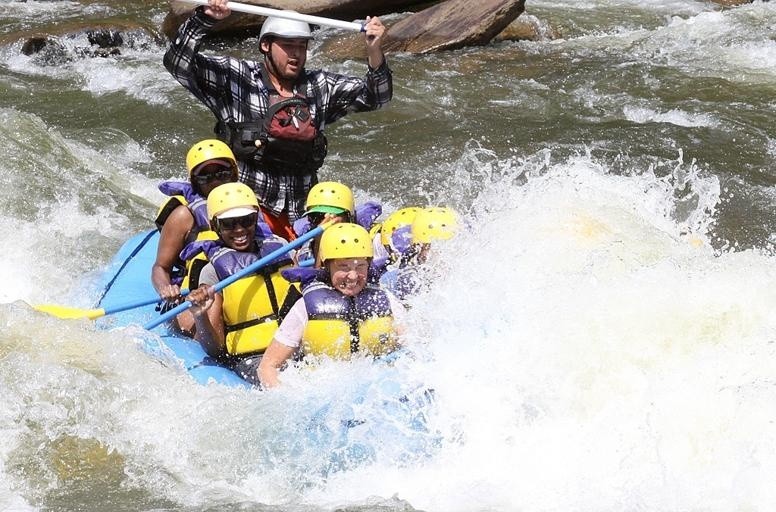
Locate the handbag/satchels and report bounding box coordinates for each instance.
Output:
[264,95,327,171]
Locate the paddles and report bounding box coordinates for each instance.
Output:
[34,287,190,321]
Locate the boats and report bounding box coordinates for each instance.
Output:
[94,229,464,469]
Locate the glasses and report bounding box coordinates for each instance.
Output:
[192,171,233,185]
[219,213,257,229]
[308,213,348,223]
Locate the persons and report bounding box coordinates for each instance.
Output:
[161,1,392,227]
[379,207,465,300]
[150,139,273,341]
[185,182,346,387]
[368,207,421,279]
[257,221,409,390]
[296,179,354,270]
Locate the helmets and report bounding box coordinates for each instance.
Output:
[382,208,423,246]
[185,140,238,181]
[207,183,259,231]
[319,223,374,266]
[303,182,355,217]
[259,10,313,53]
[411,208,464,243]
